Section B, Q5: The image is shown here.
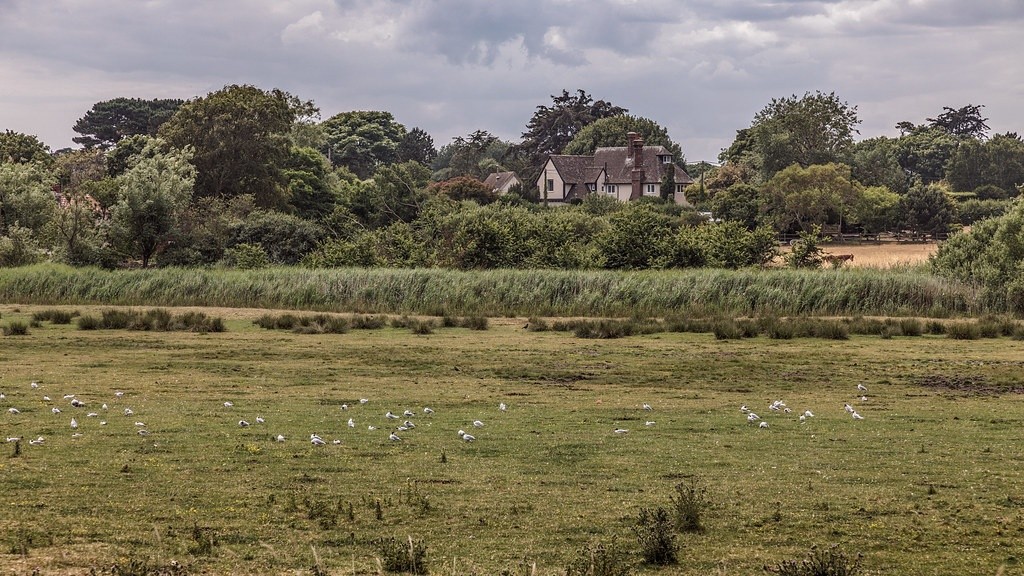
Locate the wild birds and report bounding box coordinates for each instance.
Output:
[341,398,377,431]
[424,407,435,415]
[333,439,341,445]
[614,403,657,434]
[310,431,327,447]
[223,402,234,408]
[843,380,868,420]
[256,416,265,424]
[389,426,407,441]
[278,435,286,445]
[458,429,476,443]
[239,419,250,427]
[113,392,148,439]
[499,403,507,411]
[403,410,416,430]
[0,371,107,444]
[385,411,400,421]
[474,420,484,428]
[741,399,816,429]
